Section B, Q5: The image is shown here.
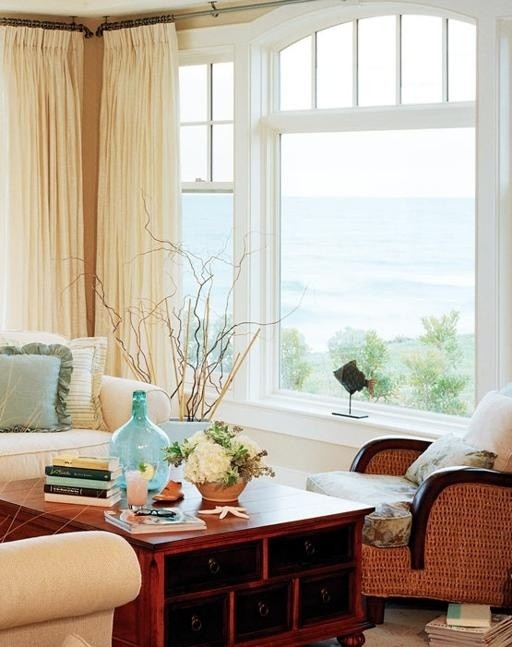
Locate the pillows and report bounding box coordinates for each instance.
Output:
[64,337,109,432]
[0,342,72,432]
[405,433,498,487]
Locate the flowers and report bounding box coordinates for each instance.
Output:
[161,421,276,486]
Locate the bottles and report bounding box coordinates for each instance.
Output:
[109,391,172,505]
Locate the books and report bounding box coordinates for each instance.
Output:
[425,603,512,647]
[43,454,123,507]
[103,506,208,534]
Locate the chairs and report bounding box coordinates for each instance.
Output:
[305,387,512,625]
[0,329,171,482]
[0,530,142,647]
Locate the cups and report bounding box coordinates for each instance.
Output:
[125,467,149,508]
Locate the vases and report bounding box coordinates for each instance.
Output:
[162,419,210,446]
[194,478,249,502]
